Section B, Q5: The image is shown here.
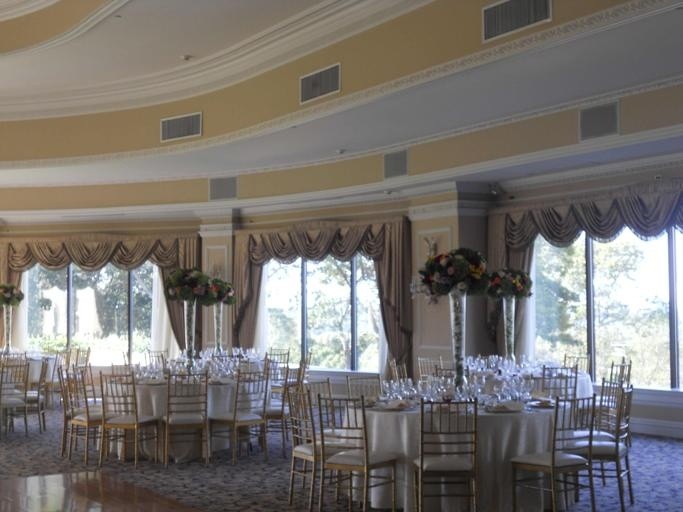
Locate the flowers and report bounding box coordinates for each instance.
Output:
[166,265,207,301]
[411,249,489,300]
[203,280,237,307]
[1,283,25,306]
[482,269,534,301]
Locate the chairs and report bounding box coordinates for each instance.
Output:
[286,351,636,511]
[2,348,308,468]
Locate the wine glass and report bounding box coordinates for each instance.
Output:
[382,355,534,411]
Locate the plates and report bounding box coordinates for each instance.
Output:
[526,400,569,408]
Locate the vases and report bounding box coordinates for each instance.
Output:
[448,291,468,385]
[183,302,198,366]
[3,306,15,355]
[212,302,224,354]
[503,294,517,364]
